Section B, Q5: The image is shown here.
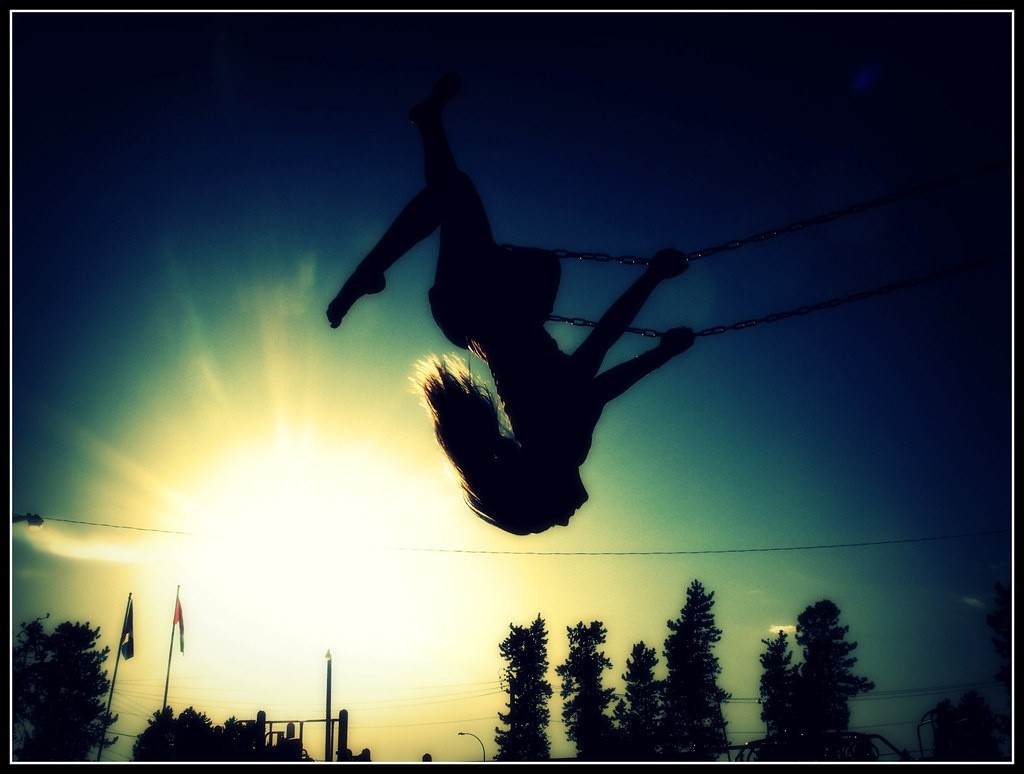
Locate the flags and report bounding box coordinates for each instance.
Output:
[175,598,184,653]
[121,600,134,660]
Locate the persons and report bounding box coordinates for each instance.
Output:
[326,74,695,537]
